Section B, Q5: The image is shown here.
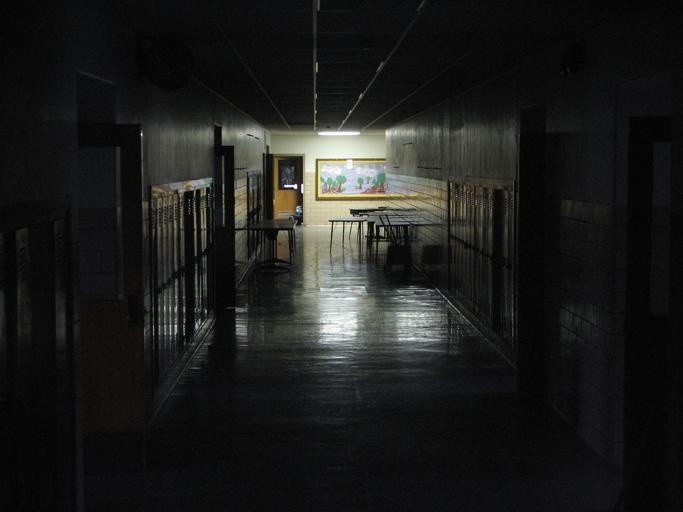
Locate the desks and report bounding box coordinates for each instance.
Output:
[328,206,410,267]
[248,219,297,266]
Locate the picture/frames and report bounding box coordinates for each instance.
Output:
[315,158,386,201]
